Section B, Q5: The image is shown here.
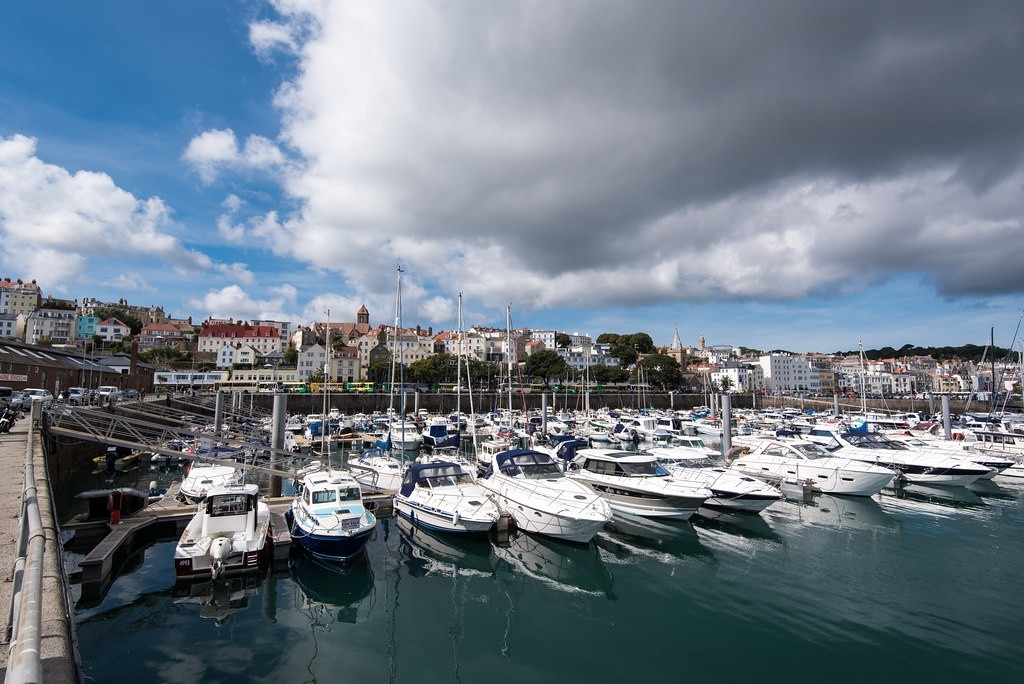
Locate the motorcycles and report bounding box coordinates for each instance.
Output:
[0,397,20,433]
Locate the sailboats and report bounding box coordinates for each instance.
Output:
[71,255,1024,583]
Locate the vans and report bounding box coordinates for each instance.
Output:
[0,386,13,412]
[96,386,118,402]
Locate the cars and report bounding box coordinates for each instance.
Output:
[118,389,140,398]
[23,388,54,406]
[11,391,33,412]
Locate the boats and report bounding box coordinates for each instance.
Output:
[871,479,992,515]
[606,507,700,553]
[287,542,375,624]
[392,509,500,573]
[489,525,619,601]
[689,504,782,545]
[998,467,1024,478]
[764,490,896,534]
[171,565,271,627]
[964,479,1011,499]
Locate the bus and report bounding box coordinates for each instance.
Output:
[551,383,578,393]
[431,383,463,393]
[213,380,258,394]
[496,383,532,394]
[310,382,344,393]
[382,382,420,394]
[346,382,377,393]
[531,384,542,393]
[627,384,651,393]
[258,382,284,393]
[283,382,310,392]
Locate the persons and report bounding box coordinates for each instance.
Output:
[62,388,71,404]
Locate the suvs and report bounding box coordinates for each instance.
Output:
[67,387,90,406]
